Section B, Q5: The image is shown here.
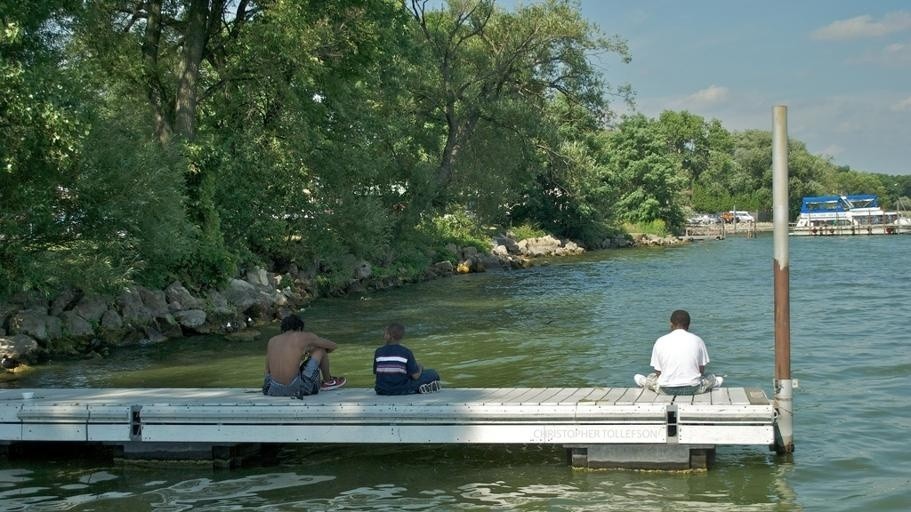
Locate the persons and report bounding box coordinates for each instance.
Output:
[633,309,725,395]
[372,322,441,395]
[261,316,348,397]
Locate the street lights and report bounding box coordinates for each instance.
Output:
[895,183,901,225]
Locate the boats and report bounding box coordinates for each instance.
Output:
[801,195,901,227]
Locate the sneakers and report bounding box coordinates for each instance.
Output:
[419,381,441,394]
[321,376,346,391]
[715,377,723,387]
[634,374,646,387]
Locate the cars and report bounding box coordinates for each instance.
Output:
[686,210,758,225]
